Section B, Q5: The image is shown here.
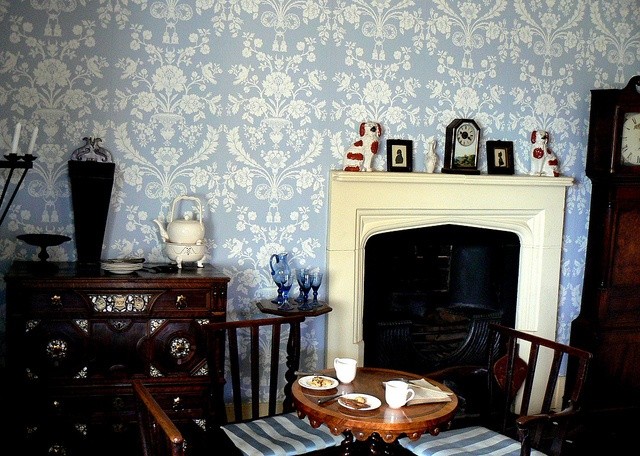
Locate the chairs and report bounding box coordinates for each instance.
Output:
[131,379,184,456]
[206,315,356,456]
[397,323,593,456]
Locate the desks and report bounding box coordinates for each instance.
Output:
[0,260,230,456]
[291,367,459,456]
[256,298,333,412]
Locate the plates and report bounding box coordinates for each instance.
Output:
[300,387,338,397]
[298,374,339,390]
[101,262,142,274]
[337,406,382,419]
[338,393,382,411]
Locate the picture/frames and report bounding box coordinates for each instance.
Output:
[486,141,514,175]
[387,139,412,172]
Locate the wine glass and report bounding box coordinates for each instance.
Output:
[278,274,294,310]
[309,272,323,306]
[298,273,313,310]
[295,268,312,302]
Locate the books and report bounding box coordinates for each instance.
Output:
[381,378,453,408]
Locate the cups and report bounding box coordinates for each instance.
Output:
[385,380,415,409]
[334,357,358,383]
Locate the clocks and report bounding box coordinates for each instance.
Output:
[562,74,640,456]
[441,119,480,175]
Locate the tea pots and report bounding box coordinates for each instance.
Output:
[270,252,291,303]
[152,195,205,244]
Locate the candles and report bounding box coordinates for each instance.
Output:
[12,122,21,153]
[28,126,38,154]
